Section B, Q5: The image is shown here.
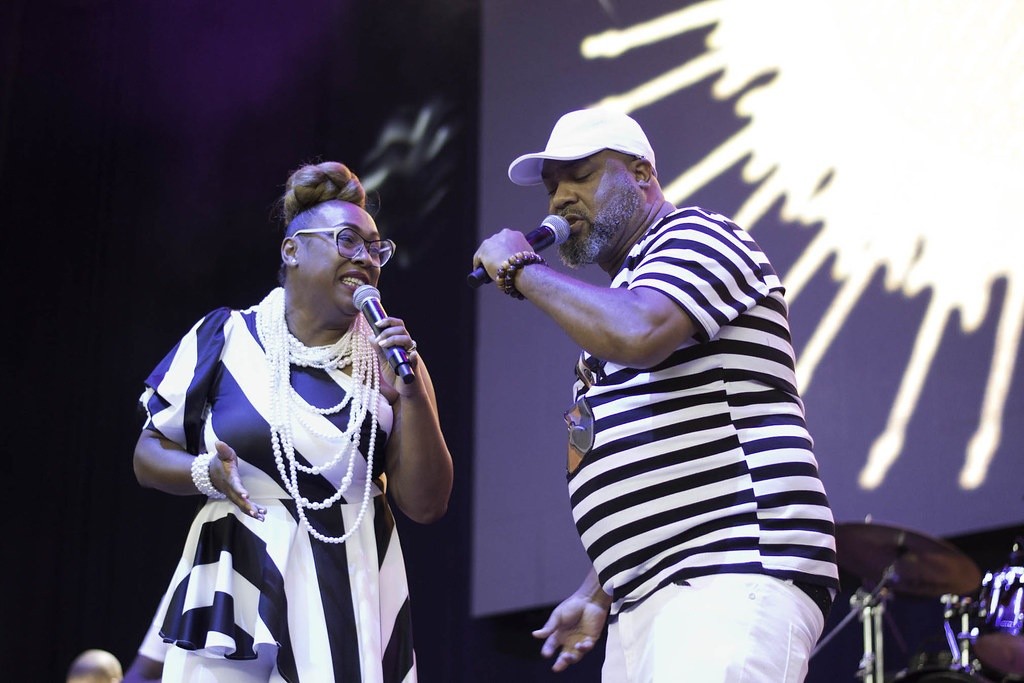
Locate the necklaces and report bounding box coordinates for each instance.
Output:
[255,287,380,544]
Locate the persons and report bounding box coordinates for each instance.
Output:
[68,650,123,683]
[474,110,839,683]
[120,161,454,683]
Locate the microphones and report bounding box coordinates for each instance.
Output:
[352,284,415,385]
[466,215,570,289]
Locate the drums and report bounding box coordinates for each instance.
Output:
[971,566,1024,674]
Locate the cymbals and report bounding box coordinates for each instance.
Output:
[835,524,981,597]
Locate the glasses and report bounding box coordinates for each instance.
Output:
[292,226,397,268]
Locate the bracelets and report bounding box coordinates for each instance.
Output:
[191,450,225,499]
[496,251,550,301]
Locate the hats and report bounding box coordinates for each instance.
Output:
[508,109,656,186]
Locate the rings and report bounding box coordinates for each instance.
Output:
[409,340,416,351]
